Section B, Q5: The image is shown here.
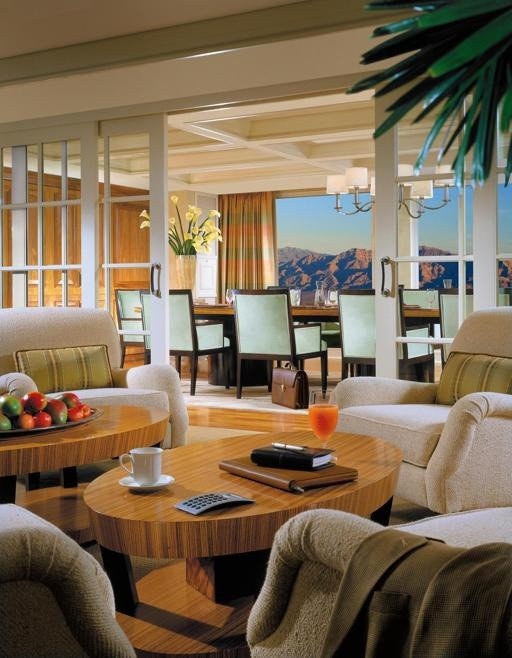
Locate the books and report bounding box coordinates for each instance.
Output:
[219,452,360,495]
[249,441,339,472]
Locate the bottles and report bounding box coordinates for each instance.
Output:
[312,280,326,309]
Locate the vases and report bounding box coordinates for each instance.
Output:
[169,250,198,293]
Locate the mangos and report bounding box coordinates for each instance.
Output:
[54,393,82,408]
[33,411,52,427]
[46,399,68,425]
[26,392,48,412]
[0,414,14,431]
[0,395,22,417]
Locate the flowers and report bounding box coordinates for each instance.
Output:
[138,195,225,256]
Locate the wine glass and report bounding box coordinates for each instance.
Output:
[328,286,337,310]
[308,388,340,450]
[225,290,235,307]
[425,287,436,309]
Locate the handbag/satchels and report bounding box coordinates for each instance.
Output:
[272,362,309,409]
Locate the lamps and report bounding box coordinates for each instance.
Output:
[324,160,455,218]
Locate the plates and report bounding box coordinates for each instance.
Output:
[120,474,174,493]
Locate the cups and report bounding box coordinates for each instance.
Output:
[120,446,163,487]
[443,278,452,288]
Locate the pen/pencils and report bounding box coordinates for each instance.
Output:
[271,441,307,451]
